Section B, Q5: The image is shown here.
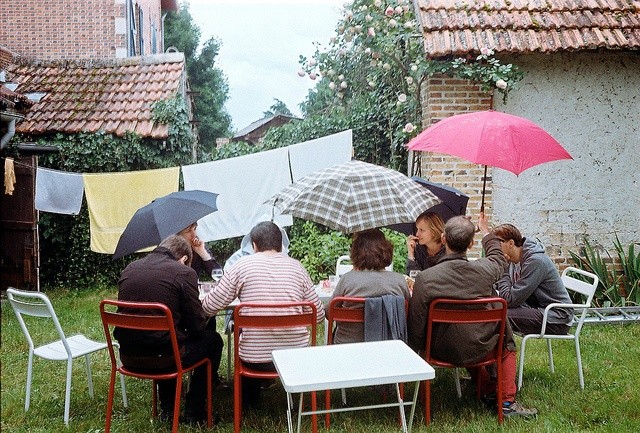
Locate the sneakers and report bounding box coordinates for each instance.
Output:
[185,413,207,422]
[160,412,176,421]
[501,402,540,420]
[260,378,276,389]
[215,378,232,390]
[480,392,495,408]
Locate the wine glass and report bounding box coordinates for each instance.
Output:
[410,270,420,281]
[211,268,223,285]
[329,275,339,295]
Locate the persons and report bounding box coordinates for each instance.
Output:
[324,228,411,344]
[406,212,447,276]
[471,223,573,386]
[408,212,538,418]
[114,236,221,427]
[205,220,326,391]
[180,221,229,393]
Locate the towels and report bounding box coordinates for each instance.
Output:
[181,146,293,244]
[31,168,84,215]
[289,128,353,183]
[84,166,179,256]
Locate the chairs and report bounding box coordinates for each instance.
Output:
[419,298,508,427]
[233,300,317,432]
[5,286,128,424]
[512,266,599,391]
[334,254,394,406]
[324,297,410,429]
[99,299,213,433]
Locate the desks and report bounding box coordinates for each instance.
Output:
[273,340,436,432]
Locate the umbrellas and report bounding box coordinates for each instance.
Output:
[112,190,219,262]
[262,160,440,236]
[383,174,470,241]
[404,109,575,231]
